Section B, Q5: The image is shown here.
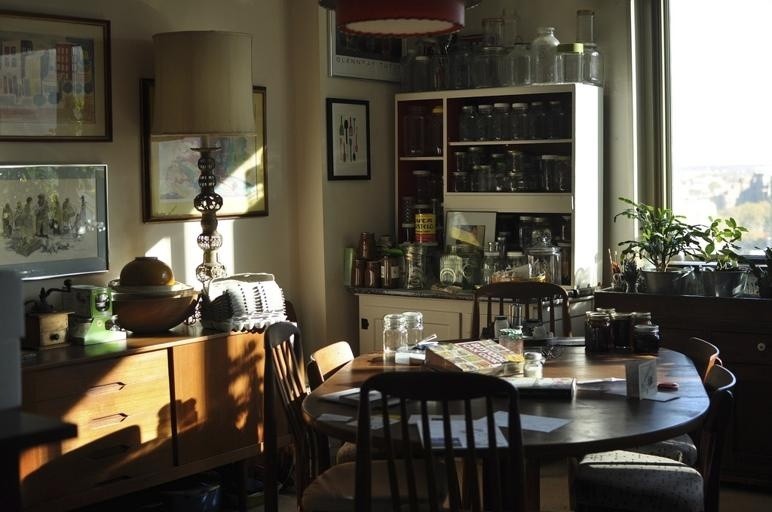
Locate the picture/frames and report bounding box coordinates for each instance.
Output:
[0,10,113,144]
[443,209,498,288]
[0,163,112,285]
[326,97,372,181]
[138,76,270,225]
[327,11,406,86]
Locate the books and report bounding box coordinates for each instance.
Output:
[420,339,525,378]
[416,415,510,452]
[318,386,414,411]
[505,376,577,402]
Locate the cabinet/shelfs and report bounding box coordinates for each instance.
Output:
[20,323,269,512]
[592,286,771,494]
[393,81,604,296]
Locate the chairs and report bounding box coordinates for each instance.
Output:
[632,332,729,463]
[304,336,418,466]
[566,362,738,510]
[469,280,577,343]
[351,368,533,511]
[265,317,454,509]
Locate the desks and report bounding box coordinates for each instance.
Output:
[312,331,713,508]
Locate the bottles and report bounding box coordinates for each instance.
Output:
[353,9,602,288]
[494,315,544,377]
[586,307,660,356]
[384,312,423,356]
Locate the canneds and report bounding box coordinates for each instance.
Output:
[414,212,437,243]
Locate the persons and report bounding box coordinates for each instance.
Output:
[0,191,89,239]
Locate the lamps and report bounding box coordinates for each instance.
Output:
[316,0,482,37]
[148,31,258,326]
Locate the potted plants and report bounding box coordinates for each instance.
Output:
[622,260,640,293]
[614,197,714,294]
[700,215,751,298]
